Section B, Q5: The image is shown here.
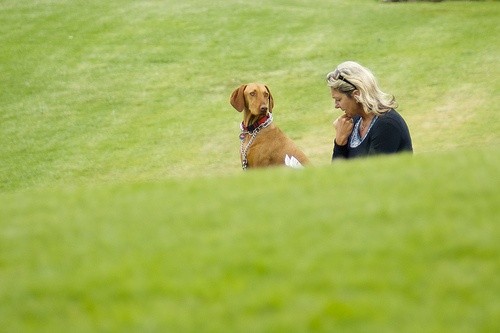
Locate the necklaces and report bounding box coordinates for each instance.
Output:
[359,110,374,131]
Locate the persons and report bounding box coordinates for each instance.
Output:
[327,60,413,163]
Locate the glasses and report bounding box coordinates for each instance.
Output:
[333,69,357,89]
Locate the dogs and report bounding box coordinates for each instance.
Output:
[230,83,311,170]
